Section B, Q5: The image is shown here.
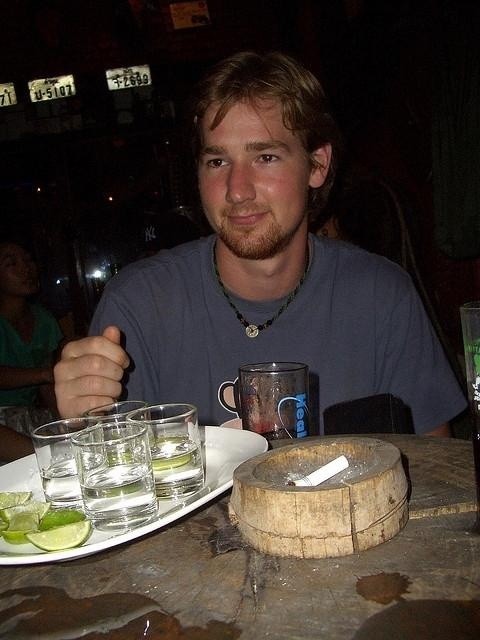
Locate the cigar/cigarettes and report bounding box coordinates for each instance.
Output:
[286,455,350,486]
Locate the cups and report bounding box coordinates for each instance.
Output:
[29,398,205,532]
[239,361,309,440]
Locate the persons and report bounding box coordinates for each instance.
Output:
[0,241,65,466]
[53,51,468,438]
[59,308,76,336]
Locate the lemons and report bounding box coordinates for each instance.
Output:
[0,491,93,551]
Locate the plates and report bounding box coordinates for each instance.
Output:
[0,421,269,567]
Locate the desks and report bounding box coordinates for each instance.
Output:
[1,432,480,639]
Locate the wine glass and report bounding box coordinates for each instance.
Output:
[459,300,480,536]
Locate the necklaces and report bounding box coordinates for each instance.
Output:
[213,237,309,338]
